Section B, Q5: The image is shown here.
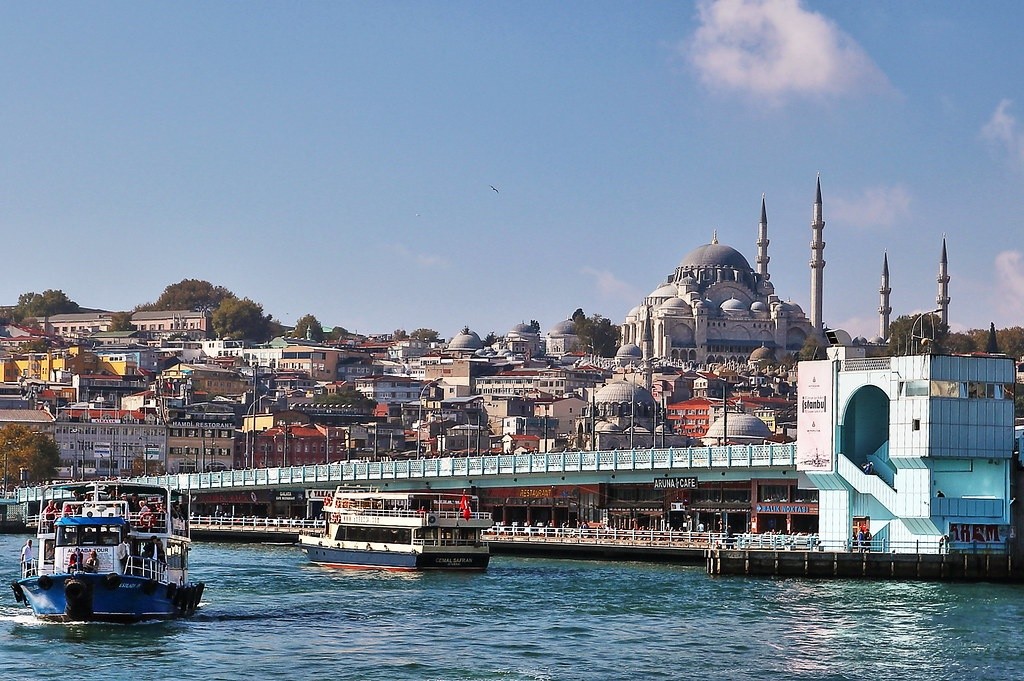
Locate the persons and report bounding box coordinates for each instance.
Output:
[852,529,872,551]
[697,522,705,532]
[144,535,166,578]
[21,540,36,576]
[68,548,99,574]
[117,538,131,574]
[862,461,874,475]
[42,490,188,527]
[190,509,326,529]
[46,542,54,559]
[496,519,687,542]
[936,490,945,498]
[726,526,734,550]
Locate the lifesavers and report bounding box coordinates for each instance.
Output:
[38,575,53,590]
[103,573,122,589]
[140,511,156,528]
[144,580,158,595]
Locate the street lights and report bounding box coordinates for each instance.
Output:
[416,376,444,460]
[245,394,268,469]
[139,435,146,477]
[630,357,660,450]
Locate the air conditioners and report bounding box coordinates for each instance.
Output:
[425,513,440,527]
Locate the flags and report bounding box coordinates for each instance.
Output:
[461,497,472,522]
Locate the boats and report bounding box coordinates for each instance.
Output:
[10,471,206,626]
[295,483,496,573]
